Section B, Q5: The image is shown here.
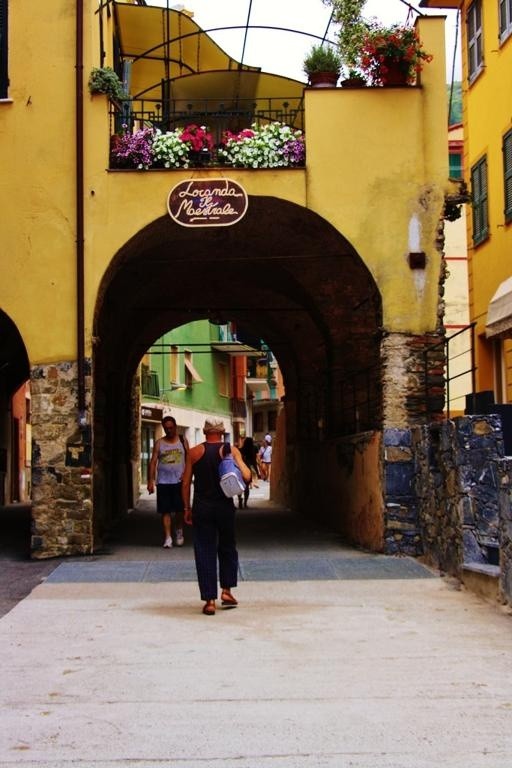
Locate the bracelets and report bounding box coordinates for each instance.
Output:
[184,507,191,511]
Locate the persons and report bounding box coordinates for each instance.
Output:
[236,436,263,510]
[182,416,253,614]
[252,431,273,481]
[147,415,189,549]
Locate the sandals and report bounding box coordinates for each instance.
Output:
[221,591,238,606]
[202,603,216,616]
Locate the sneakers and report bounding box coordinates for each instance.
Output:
[174,525,185,548]
[163,535,173,548]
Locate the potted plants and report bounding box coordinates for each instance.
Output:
[303,43,367,86]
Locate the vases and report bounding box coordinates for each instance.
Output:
[377,59,412,86]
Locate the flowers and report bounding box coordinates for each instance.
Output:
[109,120,306,171]
[357,25,433,86]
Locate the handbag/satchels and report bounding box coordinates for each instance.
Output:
[218,458,247,498]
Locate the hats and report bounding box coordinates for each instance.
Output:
[203,418,225,434]
